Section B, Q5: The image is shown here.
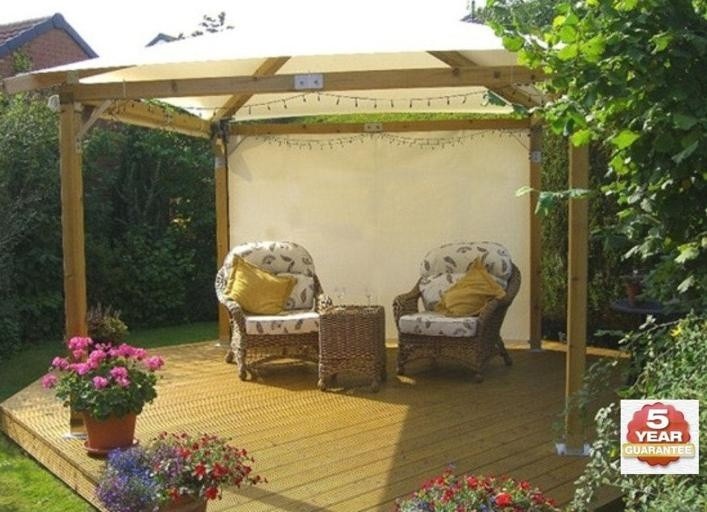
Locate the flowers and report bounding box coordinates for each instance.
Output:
[97,431,268,512]
[43,336,163,419]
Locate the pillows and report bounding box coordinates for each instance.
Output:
[225,242,313,311]
[225,254,295,315]
[419,240,511,311]
[439,256,505,317]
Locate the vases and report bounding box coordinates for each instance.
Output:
[81,413,139,457]
[160,501,206,511]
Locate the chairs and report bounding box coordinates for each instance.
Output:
[392,263,520,382]
[215,266,323,382]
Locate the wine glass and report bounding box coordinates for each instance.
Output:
[362,285,376,312]
[334,285,347,309]
